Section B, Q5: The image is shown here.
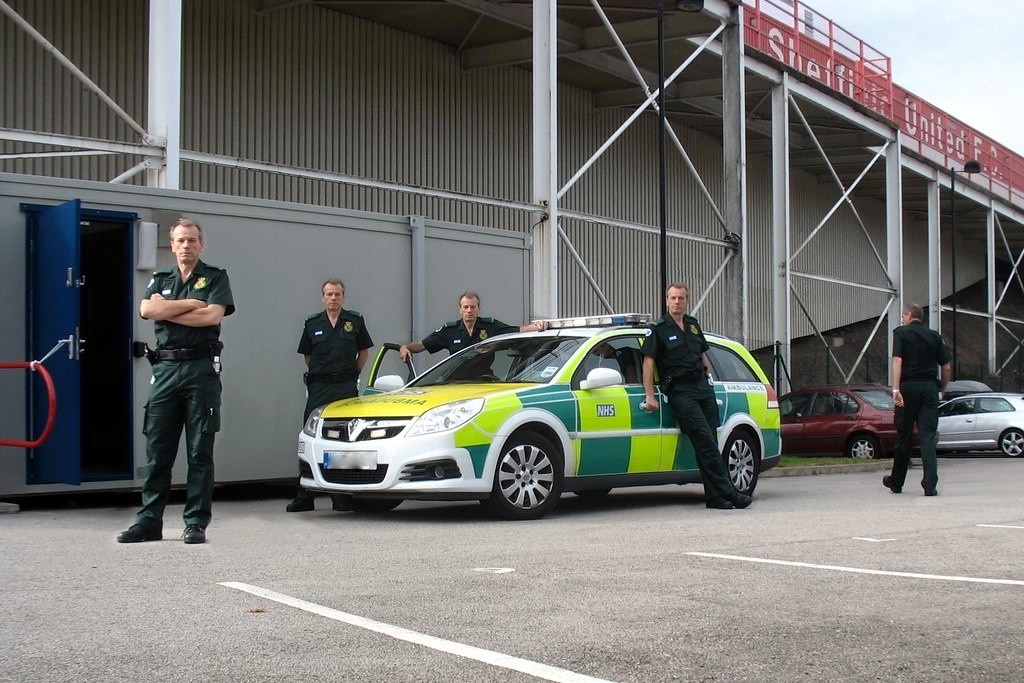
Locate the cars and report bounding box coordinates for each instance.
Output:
[779,383,940,459]
[940,380,996,411]
[935,393,1024,457]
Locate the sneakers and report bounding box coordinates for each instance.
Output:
[882,475,902,494]
[925,488,938,496]
[332,504,350,511]
[117,523,162,543]
[706,491,752,509]
[286,499,314,512]
[180,524,205,543]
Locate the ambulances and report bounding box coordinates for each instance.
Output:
[298,313,783,521]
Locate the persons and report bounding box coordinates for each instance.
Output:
[592,343,642,383]
[117,220,235,544]
[882,303,951,494]
[400,293,548,381]
[640,283,752,509]
[287,279,374,511]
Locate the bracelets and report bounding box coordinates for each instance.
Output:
[893,390,900,393]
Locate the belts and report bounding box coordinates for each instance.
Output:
[310,372,355,383]
[154,346,211,360]
[900,374,935,383]
[686,370,705,384]
